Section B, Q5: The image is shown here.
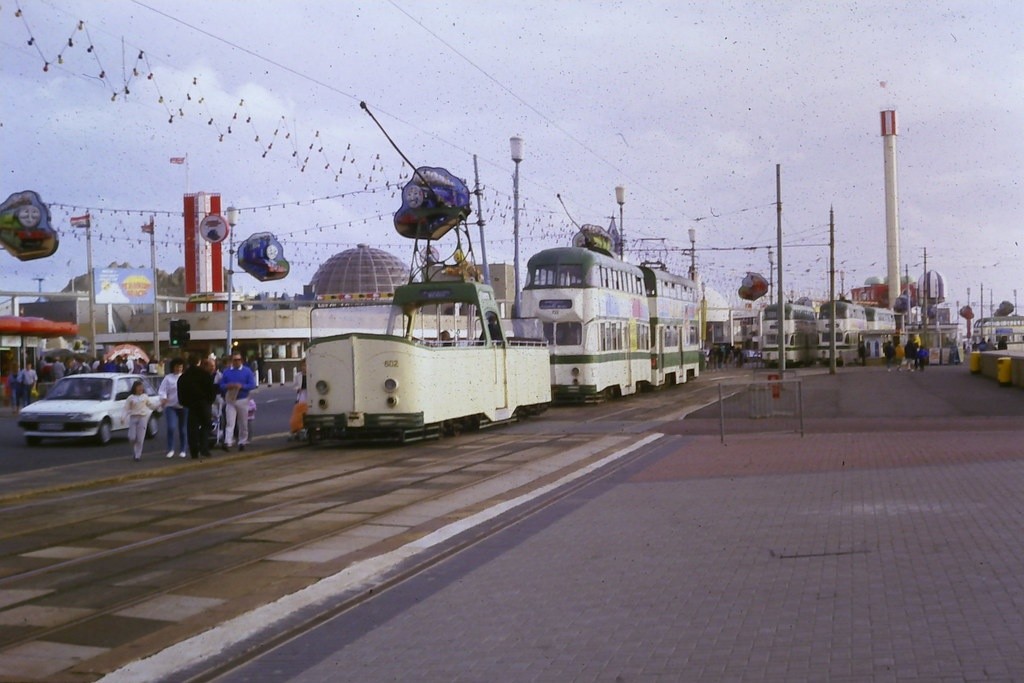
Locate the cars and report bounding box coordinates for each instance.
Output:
[19,372,164,446]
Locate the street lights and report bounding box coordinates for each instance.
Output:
[767,250,775,305]
[221,205,238,354]
[688,228,697,284]
[509,136,526,316]
[840,270,844,292]
[614,187,624,262]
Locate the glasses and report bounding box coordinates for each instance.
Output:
[233,359,241,362]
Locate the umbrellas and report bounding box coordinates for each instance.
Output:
[103,345,149,363]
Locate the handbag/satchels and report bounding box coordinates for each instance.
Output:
[31,389,38,400]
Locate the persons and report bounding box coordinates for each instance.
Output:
[441,331,452,346]
[7,370,21,413]
[120,382,158,462]
[707,343,743,369]
[177,354,216,460]
[477,316,501,346]
[97,354,157,374]
[884,342,896,372]
[38,355,100,382]
[17,363,38,407]
[286,358,308,441]
[250,353,266,382]
[858,341,866,366]
[895,339,929,372]
[978,336,1007,350]
[204,351,256,450]
[159,352,191,458]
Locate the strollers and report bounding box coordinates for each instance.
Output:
[289,389,308,442]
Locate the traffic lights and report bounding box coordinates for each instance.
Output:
[171,319,191,348]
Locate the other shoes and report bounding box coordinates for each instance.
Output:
[135,457,140,461]
[222,443,231,452]
[166,450,175,458]
[192,453,199,459]
[239,444,244,451]
[201,450,211,456]
[179,451,186,458]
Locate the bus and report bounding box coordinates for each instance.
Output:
[973,316,1024,346]
[760,301,894,368]
[520,193,701,404]
